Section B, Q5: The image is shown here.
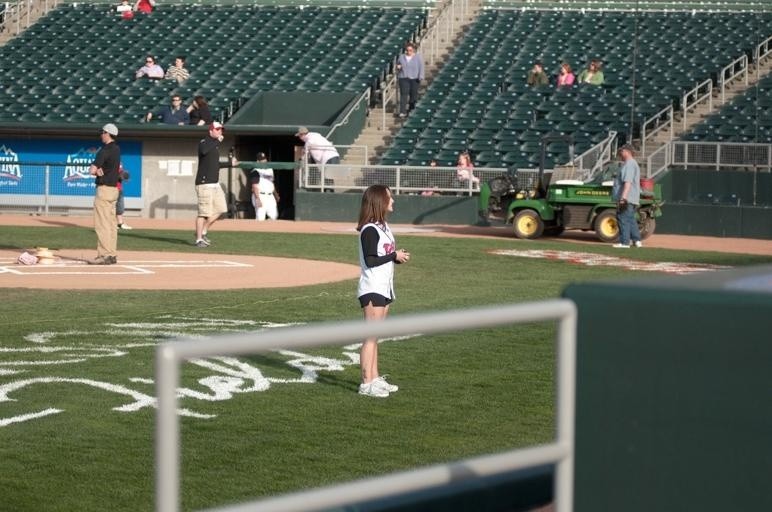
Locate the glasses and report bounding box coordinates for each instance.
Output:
[146,61,152,63]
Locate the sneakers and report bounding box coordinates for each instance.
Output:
[202,235,210,242]
[117,223,133,230]
[195,239,209,248]
[88,257,111,265]
[111,256,116,264]
[373,377,398,392]
[358,382,389,397]
[635,241,641,248]
[613,244,631,248]
[399,113,407,117]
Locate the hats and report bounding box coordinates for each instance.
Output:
[209,122,226,130]
[99,124,118,135]
[618,143,633,150]
[295,126,308,136]
[256,153,266,160]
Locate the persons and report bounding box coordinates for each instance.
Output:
[294,126,341,192]
[557,59,604,85]
[195,122,228,247]
[527,63,549,84]
[396,44,424,119]
[421,151,480,196]
[86,122,121,267]
[119,0,157,13]
[249,152,278,220]
[148,95,212,126]
[136,55,189,82]
[355,184,412,398]
[610,142,642,247]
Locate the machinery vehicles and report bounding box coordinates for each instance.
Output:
[478,163,663,244]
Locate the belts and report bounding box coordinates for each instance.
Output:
[259,192,274,195]
